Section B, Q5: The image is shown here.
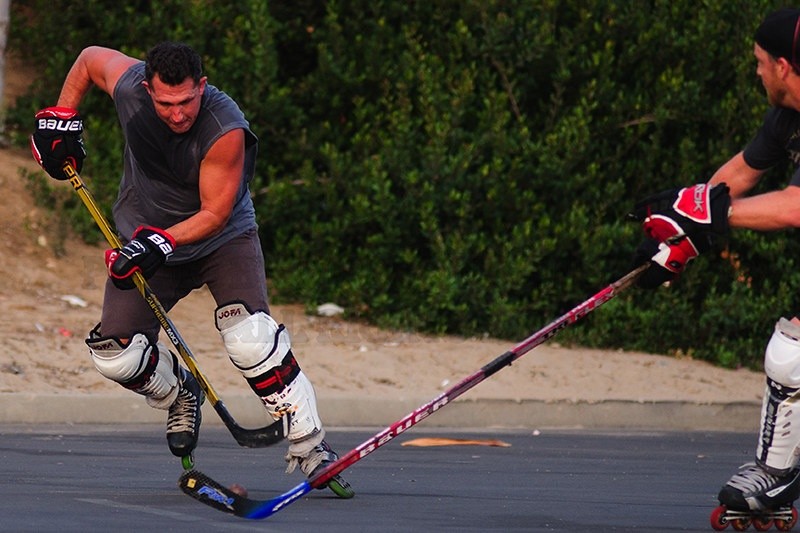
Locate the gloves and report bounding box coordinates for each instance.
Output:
[29,106,88,181]
[104,224,178,291]
[633,229,724,280]
[641,180,730,246]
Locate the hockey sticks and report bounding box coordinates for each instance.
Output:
[178,258,659,521]
[60,158,291,448]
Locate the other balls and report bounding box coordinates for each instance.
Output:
[228,484,248,498]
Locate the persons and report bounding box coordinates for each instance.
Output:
[29,41,356,498]
[628,7,800,533]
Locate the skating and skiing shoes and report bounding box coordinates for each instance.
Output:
[708,459,800,533]
[164,365,206,470]
[295,436,355,499]
